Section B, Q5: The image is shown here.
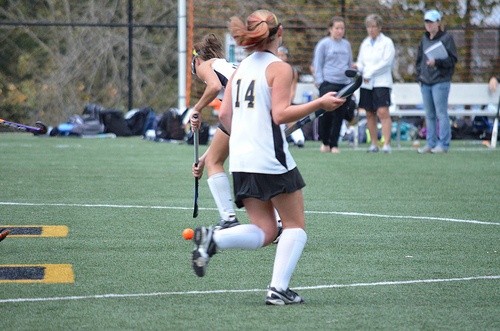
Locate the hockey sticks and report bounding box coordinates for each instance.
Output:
[191,113,199,218]
[284,68,363,138]
[482,117,498,148]
[0,118,48,134]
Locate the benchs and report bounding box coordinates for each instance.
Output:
[292,82,500,148]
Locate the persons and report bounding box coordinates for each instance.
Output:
[190,32,283,244]
[352,13,396,152]
[312,18,355,153]
[416,9,458,153]
[191,9,347,305]
[276,45,305,148]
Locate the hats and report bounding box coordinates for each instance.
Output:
[423,10,441,21]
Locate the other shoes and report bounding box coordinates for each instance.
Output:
[321,144,330,152]
[331,147,339,153]
[432,148,445,154]
[418,146,431,152]
[382,145,390,152]
[366,146,379,153]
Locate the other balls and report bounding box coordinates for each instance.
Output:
[182,227,195,240]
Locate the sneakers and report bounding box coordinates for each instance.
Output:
[271,225,282,243]
[264,285,304,306]
[191,226,218,277]
[215,217,239,231]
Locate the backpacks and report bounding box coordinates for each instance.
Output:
[49,105,208,145]
[344,117,494,143]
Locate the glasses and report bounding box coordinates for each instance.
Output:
[191,55,200,75]
